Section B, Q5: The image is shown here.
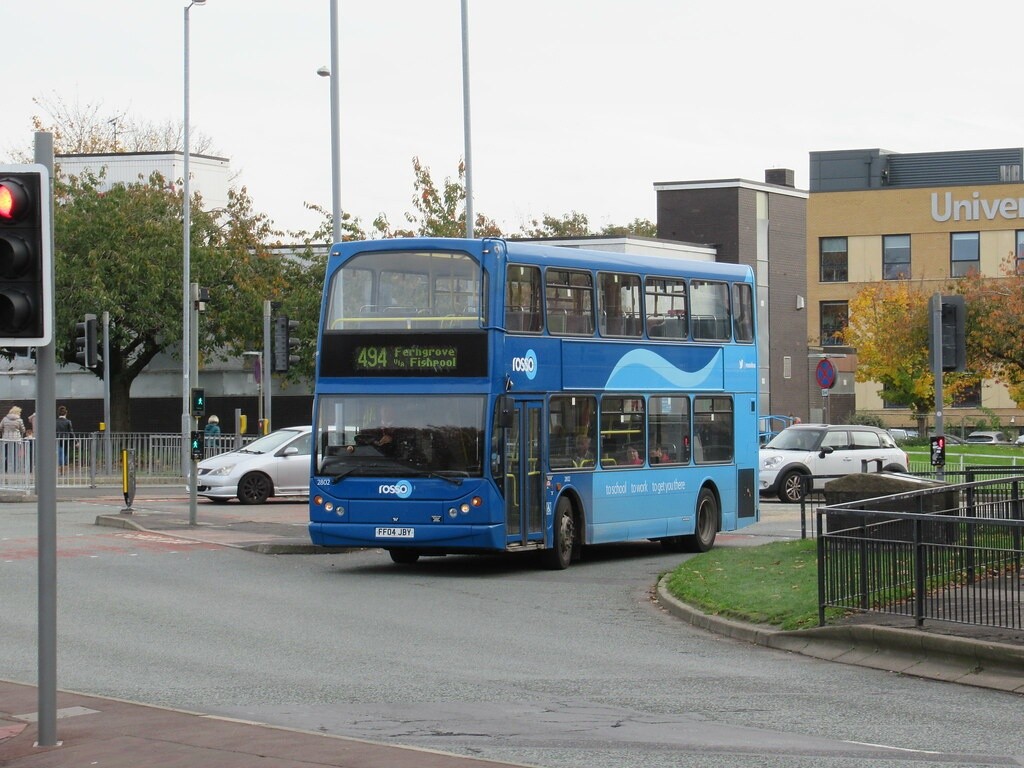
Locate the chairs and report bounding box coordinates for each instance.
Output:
[360,304,719,340]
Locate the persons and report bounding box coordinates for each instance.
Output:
[0,406,25,441]
[650,442,672,463]
[569,434,597,468]
[55,407,79,464]
[619,443,643,465]
[362,402,405,459]
[203,415,220,448]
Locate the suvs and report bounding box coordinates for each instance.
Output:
[759,424,910,504]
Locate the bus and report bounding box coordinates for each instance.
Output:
[308,239,761,570]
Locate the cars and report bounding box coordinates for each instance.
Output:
[966,430,1013,445]
[187,425,358,505]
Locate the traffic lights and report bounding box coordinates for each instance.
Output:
[191,387,205,417]
[274,314,300,372]
[75,314,98,368]
[190,430,204,460]
[0,163,52,347]
